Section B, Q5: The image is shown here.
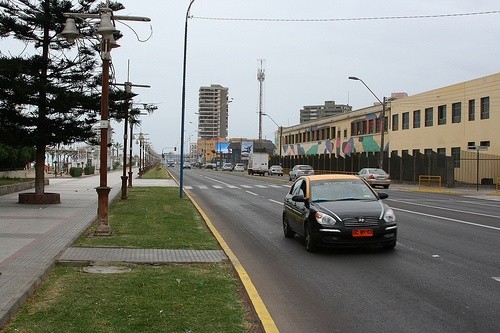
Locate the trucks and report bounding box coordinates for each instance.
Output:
[246,152,268,176]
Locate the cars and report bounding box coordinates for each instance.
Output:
[282,174,398,253]
[167,159,213,169]
[288,165,316,181]
[222,163,234,172]
[268,165,284,176]
[355,168,392,189]
[234,163,244,172]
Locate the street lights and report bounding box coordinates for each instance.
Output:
[348,77,386,169]
[108,59,161,200]
[184,98,233,171]
[60,0,151,236]
[261,114,282,166]
